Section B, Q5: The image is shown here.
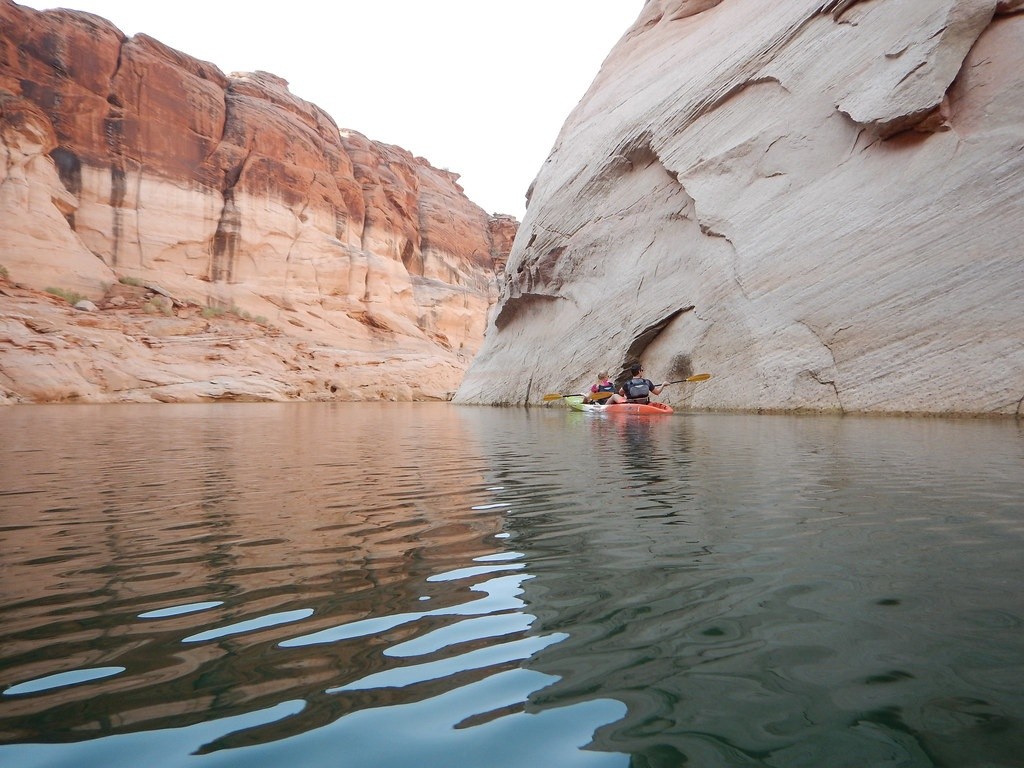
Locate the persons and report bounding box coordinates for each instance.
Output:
[583,364,670,405]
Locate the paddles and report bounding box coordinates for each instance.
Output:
[585,373,710,401]
[542,393,586,402]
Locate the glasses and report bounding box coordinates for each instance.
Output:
[608,375,609,377]
[641,370,643,372]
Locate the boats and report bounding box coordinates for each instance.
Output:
[564,395,674,415]
